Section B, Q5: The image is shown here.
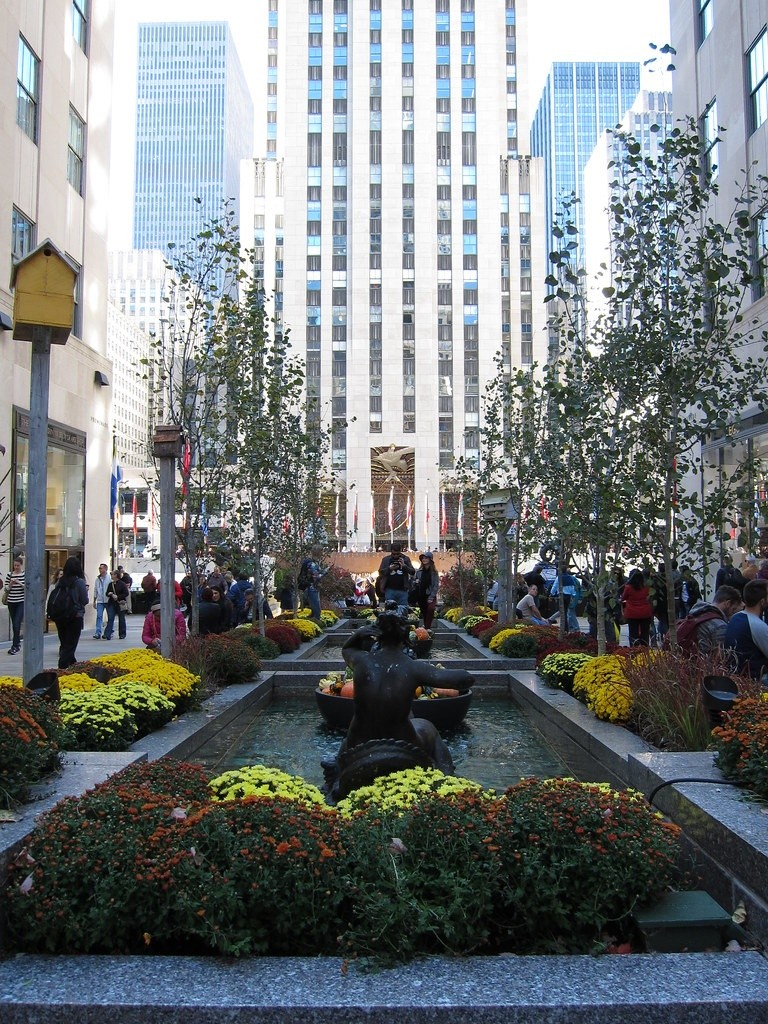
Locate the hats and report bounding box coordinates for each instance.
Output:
[419,552,434,565]
[724,553,731,559]
[356,577,364,584]
[367,577,374,585]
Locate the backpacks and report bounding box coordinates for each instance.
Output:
[662,610,724,662]
[297,560,314,590]
[47,574,82,623]
[721,567,733,585]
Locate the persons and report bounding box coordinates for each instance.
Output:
[342,544,438,552]
[715,554,768,625]
[55,556,90,669]
[120,541,204,558]
[378,542,439,630]
[353,576,385,605]
[581,559,702,646]
[487,565,580,631]
[274,578,295,613]
[689,585,742,658]
[93,564,133,639]
[141,566,274,649]
[339,600,475,776]
[724,578,768,687]
[5,557,25,654]
[302,545,332,620]
[44,569,63,619]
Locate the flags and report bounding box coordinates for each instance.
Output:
[110,435,121,517]
[182,435,191,507]
[111,493,601,535]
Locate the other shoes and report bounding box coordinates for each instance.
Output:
[120,635,126,639]
[93,634,100,639]
[8,644,22,655]
[102,634,113,640]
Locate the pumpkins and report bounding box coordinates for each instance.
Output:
[409,624,430,640]
[319,660,460,699]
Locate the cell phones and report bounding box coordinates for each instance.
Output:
[393,561,399,566]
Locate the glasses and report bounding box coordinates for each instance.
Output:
[392,552,399,555]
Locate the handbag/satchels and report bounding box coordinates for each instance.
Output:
[613,623,620,644]
[119,600,128,611]
[2,572,12,605]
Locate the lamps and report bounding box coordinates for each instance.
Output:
[92,667,112,685]
[702,674,739,727]
[26,670,60,701]
